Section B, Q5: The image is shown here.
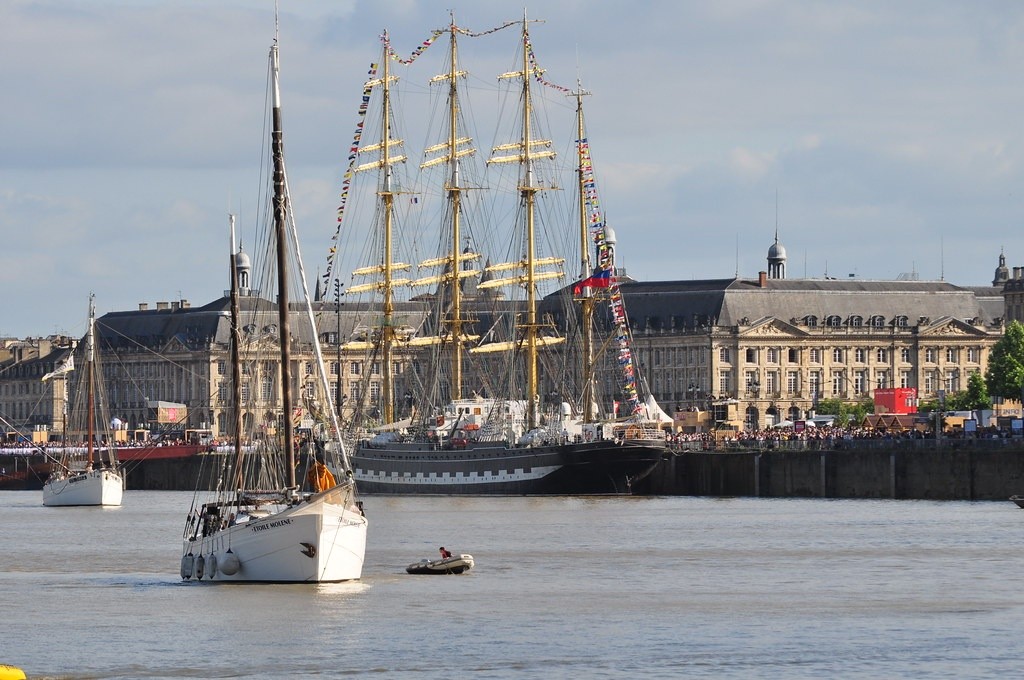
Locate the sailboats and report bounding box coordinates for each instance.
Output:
[298,6,687,497]
[179,2,369,584]
[0,289,234,506]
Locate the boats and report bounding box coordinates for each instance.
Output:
[406,553,475,574]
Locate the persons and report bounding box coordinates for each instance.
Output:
[562,429,569,441]
[0,438,235,449]
[440,547,453,559]
[666,423,1013,450]
[222,512,235,531]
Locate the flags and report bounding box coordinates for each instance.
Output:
[573,269,610,294]
